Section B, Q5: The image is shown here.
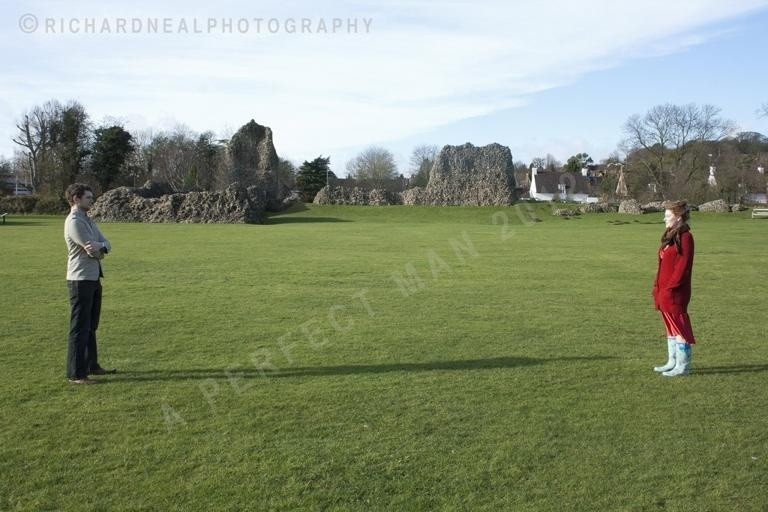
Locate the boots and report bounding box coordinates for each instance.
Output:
[653,335,675,372]
[663,342,691,377]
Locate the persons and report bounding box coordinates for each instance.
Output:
[64,183,116,384]
[653,200,696,377]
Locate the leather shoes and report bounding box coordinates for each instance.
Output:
[68,376,101,384]
[89,365,116,375]
